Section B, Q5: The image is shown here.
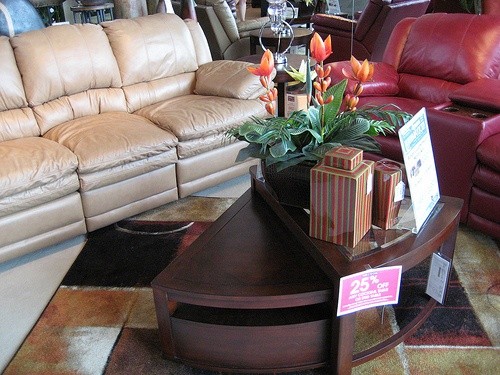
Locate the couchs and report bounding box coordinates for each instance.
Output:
[147,0,273,62]
[313,0,433,68]
[322,13,500,237]
[0,13,260,263]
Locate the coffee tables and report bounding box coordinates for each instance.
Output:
[151,178,464,375]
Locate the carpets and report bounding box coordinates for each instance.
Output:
[0,195,500,375]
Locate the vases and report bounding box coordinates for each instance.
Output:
[260,155,311,209]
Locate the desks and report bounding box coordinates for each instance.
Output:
[70,4,115,26]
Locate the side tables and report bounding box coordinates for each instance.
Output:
[235,27,317,119]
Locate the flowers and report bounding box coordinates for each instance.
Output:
[215,32,414,158]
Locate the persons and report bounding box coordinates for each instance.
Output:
[226,0,246,23]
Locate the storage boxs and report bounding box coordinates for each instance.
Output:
[310,146,407,248]
